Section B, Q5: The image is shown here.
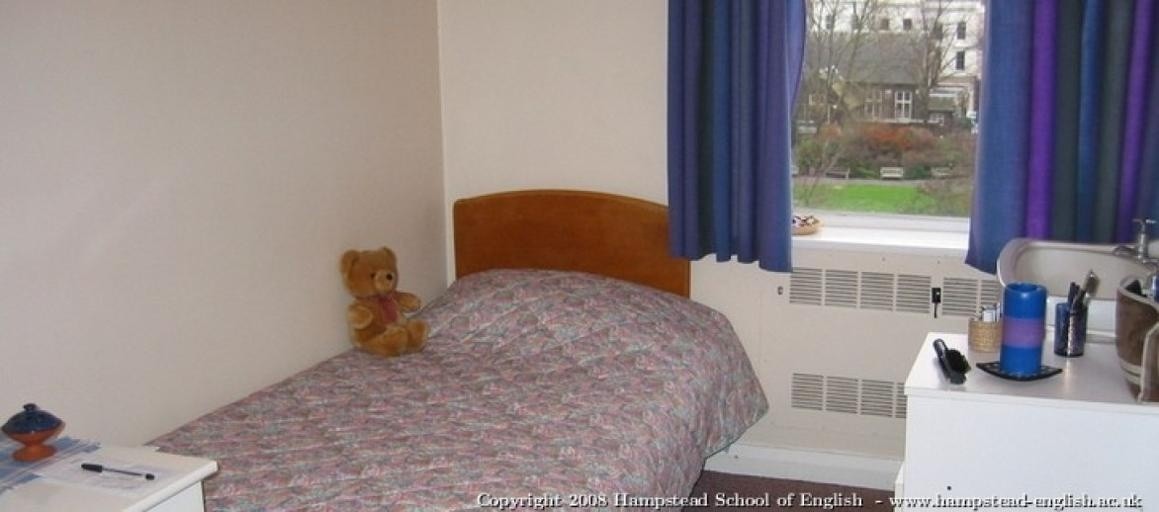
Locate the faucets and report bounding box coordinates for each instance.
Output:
[1105,242,1140,262]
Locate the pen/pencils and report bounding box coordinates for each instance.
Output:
[1059,269,1100,351]
[81,464,154,479]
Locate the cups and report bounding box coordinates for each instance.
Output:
[1000,281,1046,375]
[1052,303,1087,357]
[968,317,1004,352]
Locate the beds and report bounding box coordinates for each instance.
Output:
[142,190,772,512]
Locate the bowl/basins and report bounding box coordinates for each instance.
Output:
[792,212,824,235]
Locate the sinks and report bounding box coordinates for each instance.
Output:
[996,236,1153,301]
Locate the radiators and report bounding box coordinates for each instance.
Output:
[779,234,1010,456]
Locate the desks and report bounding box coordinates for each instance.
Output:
[1,426,223,512]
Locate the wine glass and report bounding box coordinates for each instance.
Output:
[0,426,61,462]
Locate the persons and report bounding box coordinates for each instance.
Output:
[341,246,429,358]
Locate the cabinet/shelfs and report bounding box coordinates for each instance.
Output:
[885,319,1159,512]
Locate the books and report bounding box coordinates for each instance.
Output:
[34,452,179,494]
